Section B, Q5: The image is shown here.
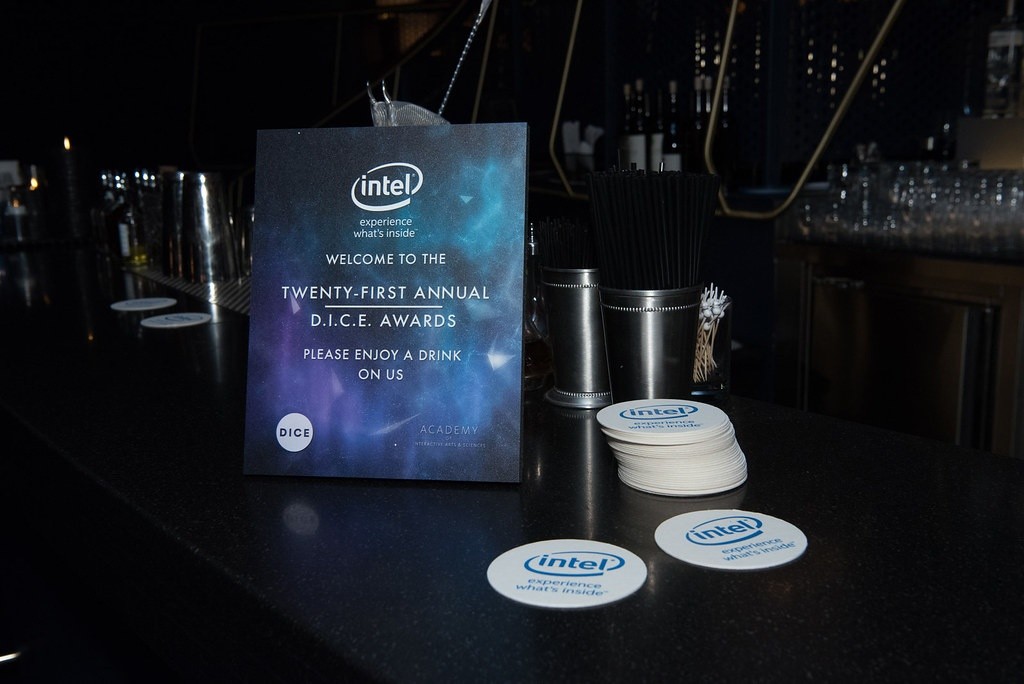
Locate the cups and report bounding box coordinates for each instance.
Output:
[182,173,237,284]
[541,265,613,410]
[692,296,736,399]
[162,171,187,280]
[596,283,705,404]
[821,160,1024,254]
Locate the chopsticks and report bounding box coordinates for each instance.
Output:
[538,216,590,268]
[587,161,715,290]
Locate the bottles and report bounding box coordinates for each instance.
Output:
[617,74,689,174]
